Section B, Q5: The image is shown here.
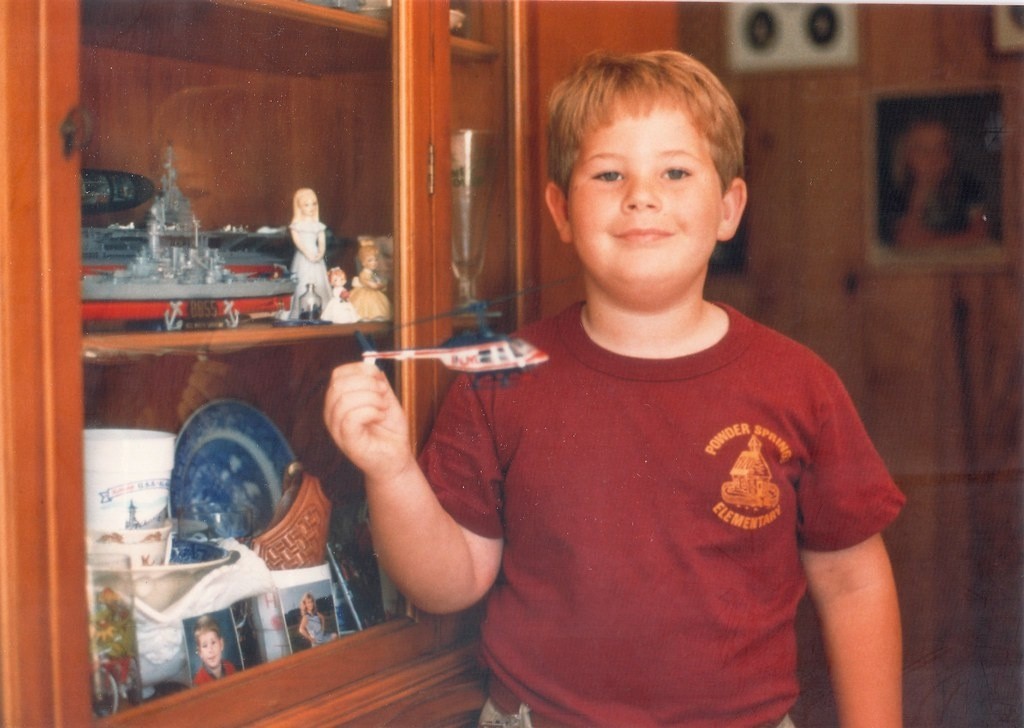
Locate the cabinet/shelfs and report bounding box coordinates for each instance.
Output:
[0,0,541,728]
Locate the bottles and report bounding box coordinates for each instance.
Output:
[299,284,321,320]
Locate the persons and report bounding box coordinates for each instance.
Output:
[270,187,390,324]
[192,616,237,685]
[298,592,339,648]
[321,51,904,728]
[889,114,991,250]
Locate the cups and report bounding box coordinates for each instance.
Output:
[82,428,176,529]
[85,520,171,565]
[84,554,141,718]
[179,502,252,548]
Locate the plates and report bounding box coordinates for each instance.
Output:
[167,398,296,550]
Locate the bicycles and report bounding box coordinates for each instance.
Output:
[91,617,130,718]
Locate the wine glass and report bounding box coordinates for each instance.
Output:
[450,129,503,318]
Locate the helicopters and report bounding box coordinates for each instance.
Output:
[354,274,579,389]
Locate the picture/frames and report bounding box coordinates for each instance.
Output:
[861,80,1017,277]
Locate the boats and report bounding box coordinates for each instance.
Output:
[78,140,346,331]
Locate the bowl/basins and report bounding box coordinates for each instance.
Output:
[89,539,238,612]
[136,624,187,697]
